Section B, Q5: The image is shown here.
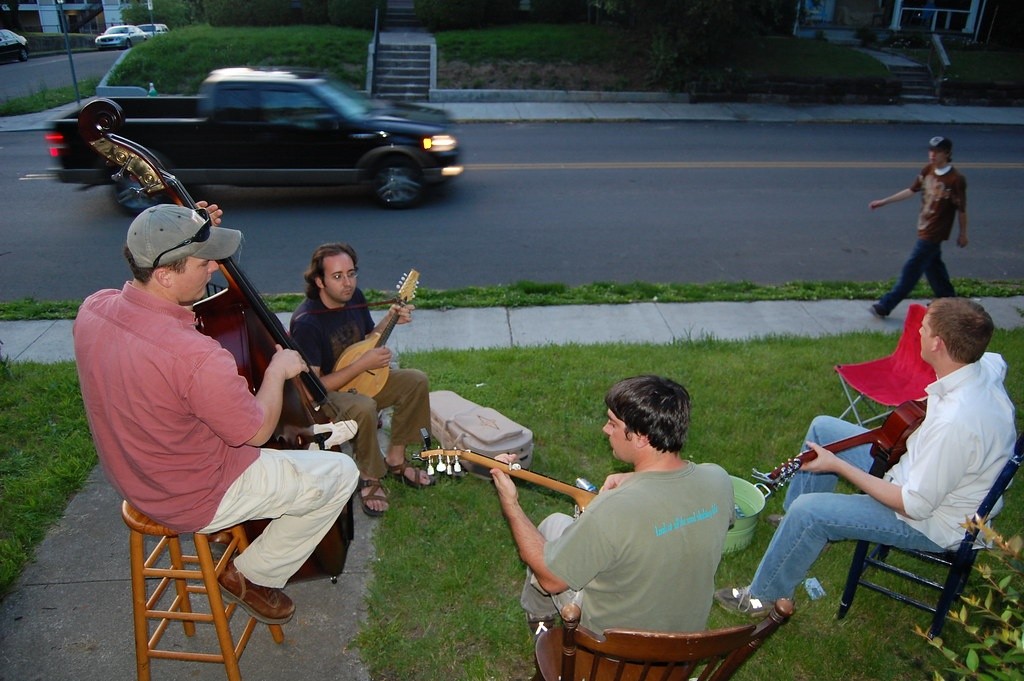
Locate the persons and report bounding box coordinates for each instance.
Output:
[291,242,435,516]
[72,201,359,624]
[714,297,1017,617]
[490,374,736,636]
[868,137,969,318]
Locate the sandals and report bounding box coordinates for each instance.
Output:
[383,457,437,489]
[357,476,390,517]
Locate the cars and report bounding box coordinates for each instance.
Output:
[0,29,29,62]
[95,25,145,51]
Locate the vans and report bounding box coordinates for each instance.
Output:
[136,24,170,38]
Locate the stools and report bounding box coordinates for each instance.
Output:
[121,499,285,681]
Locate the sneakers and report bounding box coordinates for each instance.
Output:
[766,513,785,528]
[217,561,296,625]
[714,584,796,618]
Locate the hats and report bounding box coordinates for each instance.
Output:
[126,204,242,268]
[925,135,952,151]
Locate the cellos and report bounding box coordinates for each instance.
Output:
[75,97,354,581]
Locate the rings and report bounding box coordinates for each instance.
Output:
[409,309,412,314]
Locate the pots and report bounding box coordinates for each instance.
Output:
[722,474,771,555]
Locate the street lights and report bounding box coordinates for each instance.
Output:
[57,0,80,103]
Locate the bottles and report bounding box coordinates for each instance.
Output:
[575,477,598,495]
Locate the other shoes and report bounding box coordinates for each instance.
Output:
[871,304,885,319]
[525,609,555,634]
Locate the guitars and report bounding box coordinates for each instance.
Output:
[419,445,599,511]
[768,400,926,490]
[333,269,420,394]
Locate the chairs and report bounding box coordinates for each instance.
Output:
[909,4,936,28]
[530,597,795,681]
[837,434,1024,635]
[872,4,894,26]
[834,304,937,428]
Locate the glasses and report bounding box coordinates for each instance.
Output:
[153,208,212,268]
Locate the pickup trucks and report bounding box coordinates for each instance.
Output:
[45,67,463,210]
